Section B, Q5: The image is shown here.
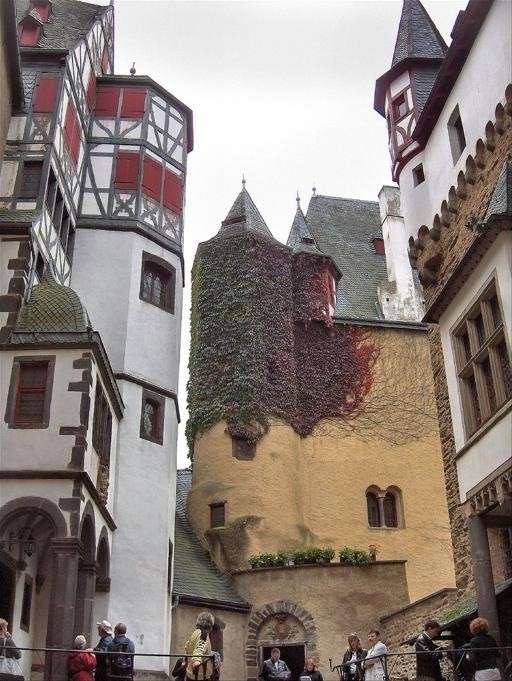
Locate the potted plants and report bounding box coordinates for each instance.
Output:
[248,545,381,568]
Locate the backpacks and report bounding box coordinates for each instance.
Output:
[111,637,134,676]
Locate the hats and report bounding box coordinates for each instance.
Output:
[96,620,114,634]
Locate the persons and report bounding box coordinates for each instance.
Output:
[64,634,97,681]
[93,620,114,680]
[0,618,26,681]
[464,615,512,681]
[342,631,369,681]
[413,622,451,680]
[301,659,323,681]
[359,629,387,681]
[107,623,135,681]
[183,612,219,681]
[262,649,292,680]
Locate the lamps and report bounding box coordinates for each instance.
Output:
[0,524,37,558]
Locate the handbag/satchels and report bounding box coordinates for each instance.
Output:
[0,638,24,681]
